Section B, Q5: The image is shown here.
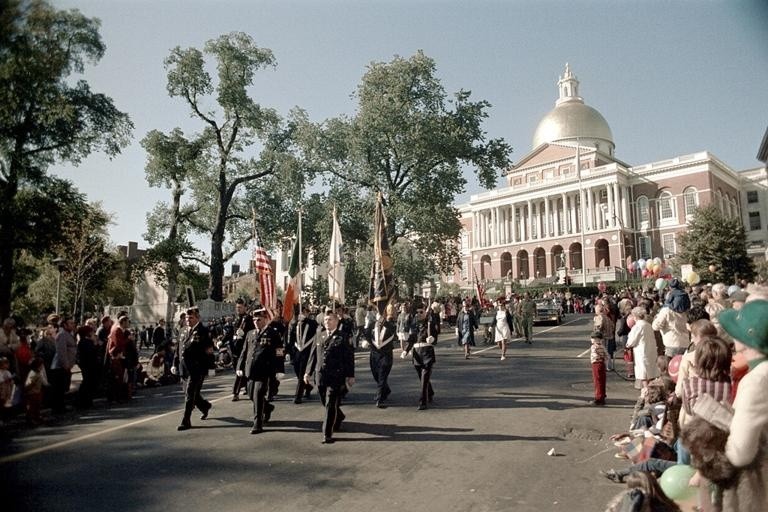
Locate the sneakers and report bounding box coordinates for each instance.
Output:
[249,425,263,434]
[333,413,345,432]
[230,380,280,401]
[465,353,470,359]
[321,435,331,443]
[262,404,275,422]
[500,355,505,361]
[294,395,300,404]
[587,396,607,406]
[200,402,212,420]
[177,425,190,430]
[304,386,313,397]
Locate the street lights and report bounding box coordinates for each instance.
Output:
[50,251,70,316]
[106,294,116,317]
[506,269,512,281]
[94,304,102,329]
[520,272,527,288]
[599,203,627,270]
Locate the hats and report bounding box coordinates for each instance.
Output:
[78,326,93,336]
[253,307,268,317]
[415,303,427,310]
[591,330,603,338]
[716,297,768,361]
[663,287,692,313]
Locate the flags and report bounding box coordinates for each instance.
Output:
[573,147,581,184]
[253,227,278,310]
[327,214,346,306]
[368,201,397,316]
[282,223,305,324]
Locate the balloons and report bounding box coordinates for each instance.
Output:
[627,257,672,290]
[709,265,715,273]
[686,270,698,285]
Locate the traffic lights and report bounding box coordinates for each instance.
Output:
[562,276,567,286]
[568,276,573,285]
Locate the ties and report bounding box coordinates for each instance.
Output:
[327,331,331,338]
[257,329,262,336]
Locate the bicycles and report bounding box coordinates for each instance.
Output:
[613,341,695,383]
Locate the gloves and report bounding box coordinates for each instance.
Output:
[236,370,244,377]
[275,372,285,381]
[170,366,176,375]
[208,369,216,376]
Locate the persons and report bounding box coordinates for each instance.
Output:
[0,281,768,511]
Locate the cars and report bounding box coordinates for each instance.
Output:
[531,298,563,326]
